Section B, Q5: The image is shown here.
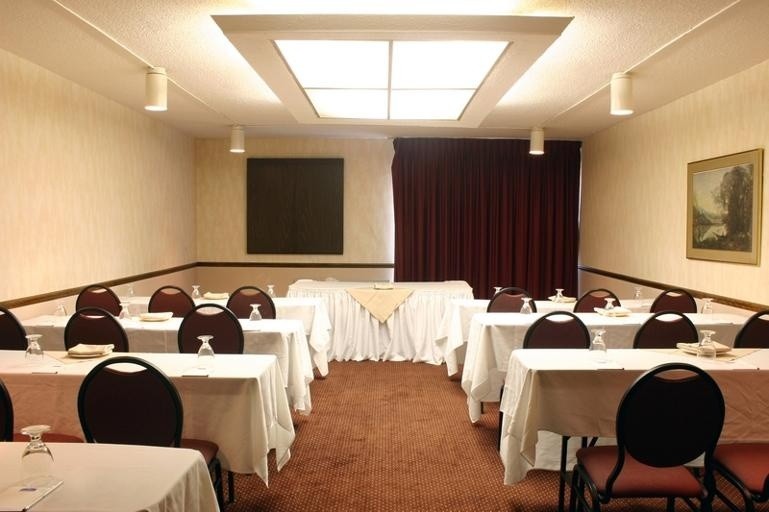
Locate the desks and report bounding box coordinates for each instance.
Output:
[285,278,475,366]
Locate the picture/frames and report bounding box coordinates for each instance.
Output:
[685,147,764,268]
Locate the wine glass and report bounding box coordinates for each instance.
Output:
[128,287,135,298]
[249,304,262,321]
[119,303,132,320]
[520,298,532,315]
[701,298,713,314]
[53,302,65,320]
[697,330,716,362]
[589,329,606,352]
[604,298,616,309]
[632,285,641,300]
[493,287,502,296]
[197,336,214,368]
[555,289,564,303]
[192,286,201,299]
[20,425,54,482]
[267,285,274,297]
[25,334,43,362]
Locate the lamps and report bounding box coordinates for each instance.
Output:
[610,72,635,116]
[529,126,545,156]
[144,67,168,112]
[229,125,245,154]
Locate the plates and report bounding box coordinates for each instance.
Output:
[597,312,628,316]
[144,319,166,321]
[70,354,101,357]
[683,350,725,356]
[375,288,393,290]
